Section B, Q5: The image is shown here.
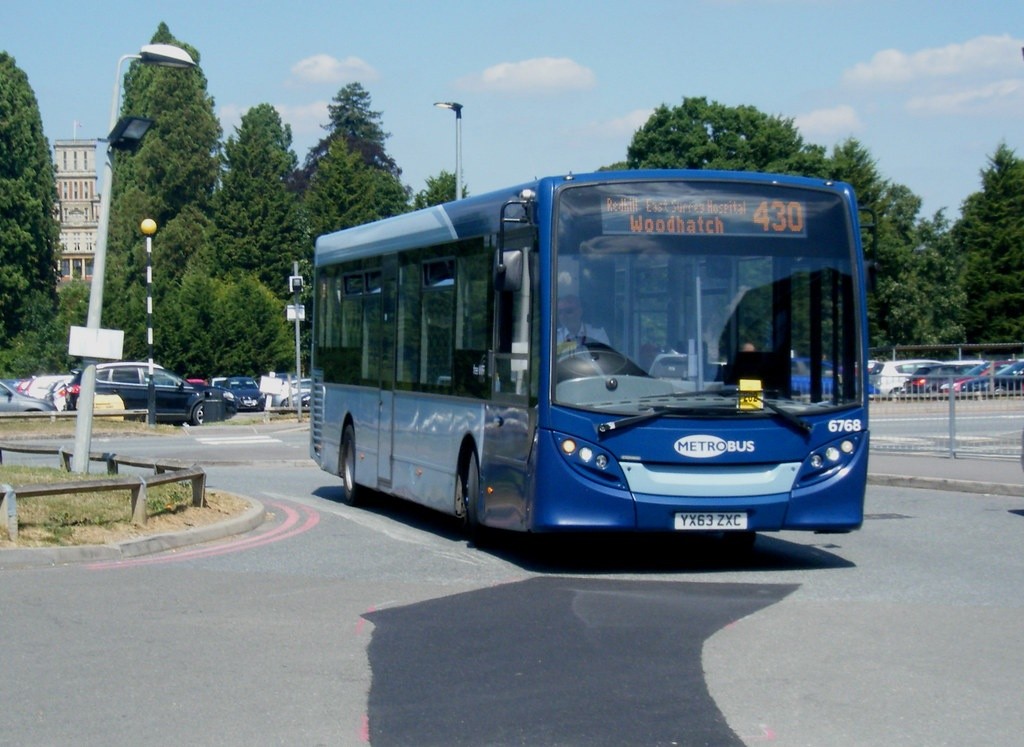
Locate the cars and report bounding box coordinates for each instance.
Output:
[471,339,1024,401]
[0,372,312,413]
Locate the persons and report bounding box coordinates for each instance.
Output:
[556,294,611,356]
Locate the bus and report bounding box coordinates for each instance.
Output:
[306,166,870,549]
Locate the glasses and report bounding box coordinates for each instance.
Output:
[558,307,576,314]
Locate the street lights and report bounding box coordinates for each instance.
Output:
[433,101,465,351]
[68,41,198,475]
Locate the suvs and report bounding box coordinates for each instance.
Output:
[66,360,238,427]
